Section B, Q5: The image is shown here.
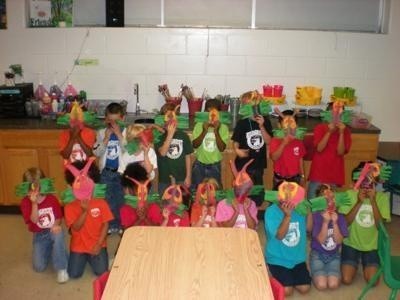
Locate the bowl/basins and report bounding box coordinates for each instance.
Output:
[295,110,307,118]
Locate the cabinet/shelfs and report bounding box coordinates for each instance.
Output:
[0,131,71,207]
[220,133,380,212]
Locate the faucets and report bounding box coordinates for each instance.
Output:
[133,82,141,116]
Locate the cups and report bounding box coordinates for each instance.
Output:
[5,71,15,87]
[10,64,23,84]
[231,98,240,113]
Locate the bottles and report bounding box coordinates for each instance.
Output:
[25,83,87,117]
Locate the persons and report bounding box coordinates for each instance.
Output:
[161,187,191,226]
[118,122,158,197]
[306,183,348,289]
[338,162,391,284]
[191,99,228,191]
[217,173,258,228]
[17,167,69,283]
[92,104,127,234]
[191,179,218,227]
[268,110,307,204]
[308,101,351,200]
[64,160,111,280]
[154,103,191,198]
[230,90,272,207]
[264,182,314,295]
[120,162,161,226]
[59,100,102,186]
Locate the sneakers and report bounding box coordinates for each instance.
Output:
[57,269,70,283]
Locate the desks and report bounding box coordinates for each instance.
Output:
[100,225,275,300]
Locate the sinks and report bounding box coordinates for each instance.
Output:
[133,118,155,125]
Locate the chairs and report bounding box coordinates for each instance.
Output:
[357,218,400,300]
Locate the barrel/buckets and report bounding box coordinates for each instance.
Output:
[165,96,183,114]
[229,98,241,115]
[220,104,229,113]
[188,97,203,116]
